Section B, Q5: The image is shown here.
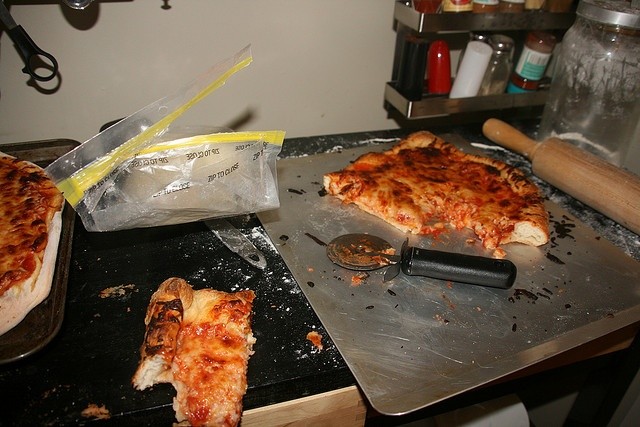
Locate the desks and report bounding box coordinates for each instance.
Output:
[0,118,640,426]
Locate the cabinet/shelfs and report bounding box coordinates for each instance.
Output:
[381,1,578,132]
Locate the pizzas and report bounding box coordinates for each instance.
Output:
[318,129,551,246]
[1,152,67,336]
[132,277,256,427]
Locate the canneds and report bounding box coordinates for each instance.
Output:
[513,31,555,82]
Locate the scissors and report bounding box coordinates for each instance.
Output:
[1,1,62,82]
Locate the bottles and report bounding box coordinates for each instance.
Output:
[479,34,515,96]
[426,38,452,95]
[447,41,492,99]
[390,19,427,100]
[507,32,554,94]
